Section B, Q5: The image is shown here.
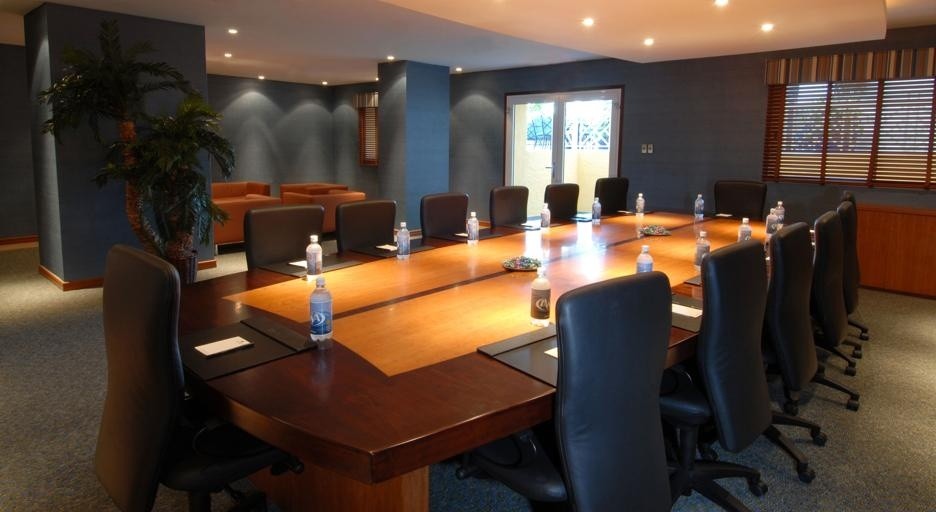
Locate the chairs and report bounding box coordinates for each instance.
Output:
[94,242,274,512]
[489,185,529,229]
[594,178,629,216]
[658,180,868,511]
[543,182,580,222]
[334,198,399,253]
[419,192,471,240]
[242,203,328,272]
[455,270,675,512]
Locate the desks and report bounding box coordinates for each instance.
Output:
[179,208,789,512]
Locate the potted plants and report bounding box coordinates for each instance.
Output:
[36,18,236,286]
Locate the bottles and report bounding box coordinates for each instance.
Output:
[307,278,333,341]
[396,222,410,260]
[468,212,479,244]
[530,267,552,327]
[305,234,323,280]
[539,193,785,276]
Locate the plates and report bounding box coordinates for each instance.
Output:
[502,261,541,271]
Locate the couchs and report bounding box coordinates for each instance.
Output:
[210,180,367,252]
